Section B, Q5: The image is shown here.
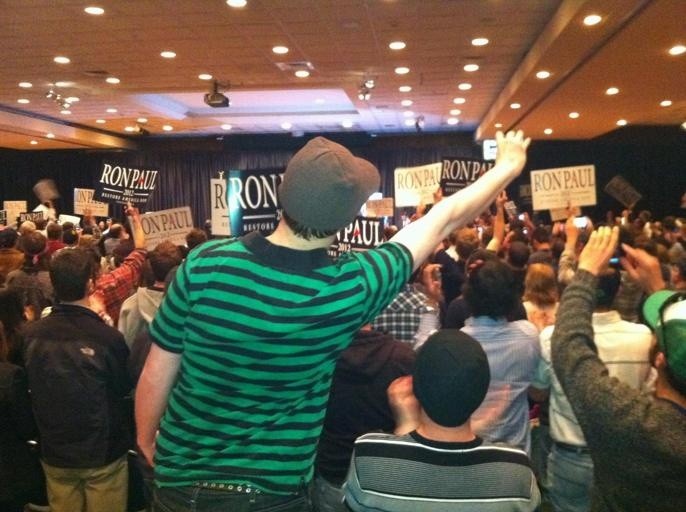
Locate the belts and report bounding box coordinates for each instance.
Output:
[194,481,260,493]
[556,442,589,455]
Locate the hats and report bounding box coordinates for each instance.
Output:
[279,135,381,230]
[643,290,685,388]
[413,330,491,425]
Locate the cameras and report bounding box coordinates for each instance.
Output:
[573,217,587,229]
[483,139,496,161]
[608,240,626,271]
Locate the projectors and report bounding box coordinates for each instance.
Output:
[204,93,229,108]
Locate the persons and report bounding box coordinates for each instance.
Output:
[132,129,531,511]
[0,202,209,511]
[311,190,686,512]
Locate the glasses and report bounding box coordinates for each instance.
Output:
[658,294,685,368]
